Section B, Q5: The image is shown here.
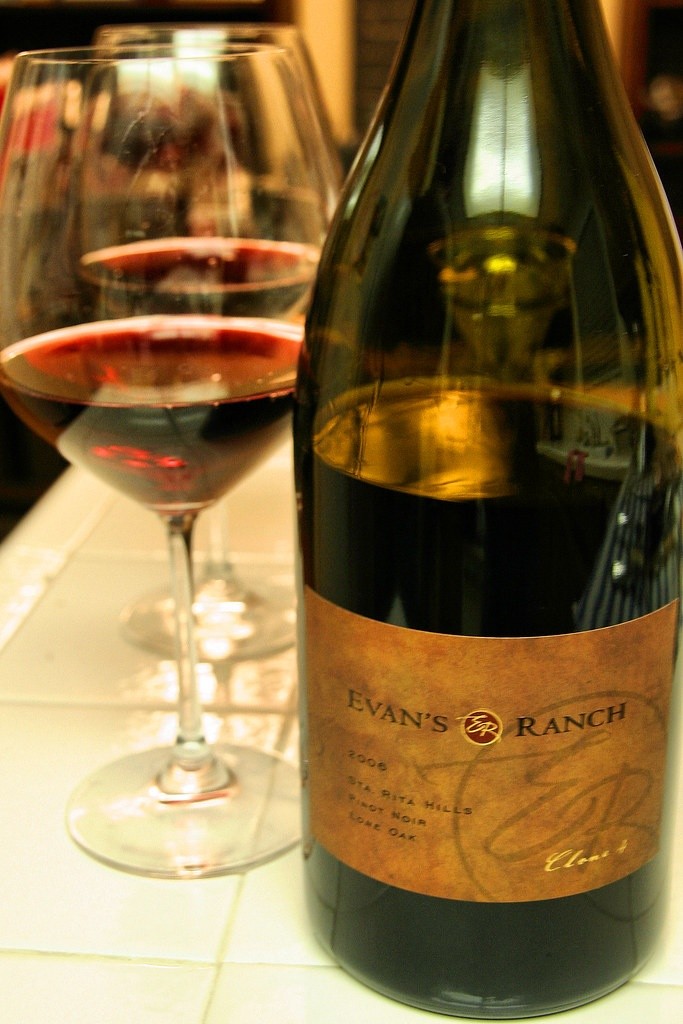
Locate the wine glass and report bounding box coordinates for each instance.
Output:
[0,24,340,880]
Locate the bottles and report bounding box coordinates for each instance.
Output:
[293,2,683,1018]
